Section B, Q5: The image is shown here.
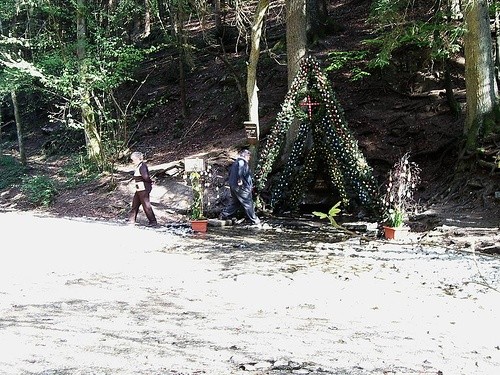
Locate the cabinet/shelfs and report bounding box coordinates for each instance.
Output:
[244,120,258,144]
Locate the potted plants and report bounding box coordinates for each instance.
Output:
[382,210,411,240]
[188,157,209,232]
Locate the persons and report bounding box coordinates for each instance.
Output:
[126,152,158,227]
[218,148,264,225]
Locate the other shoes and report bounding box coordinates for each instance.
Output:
[147,220,157,226]
[217,214,230,220]
[251,220,266,225]
[125,224,135,228]
